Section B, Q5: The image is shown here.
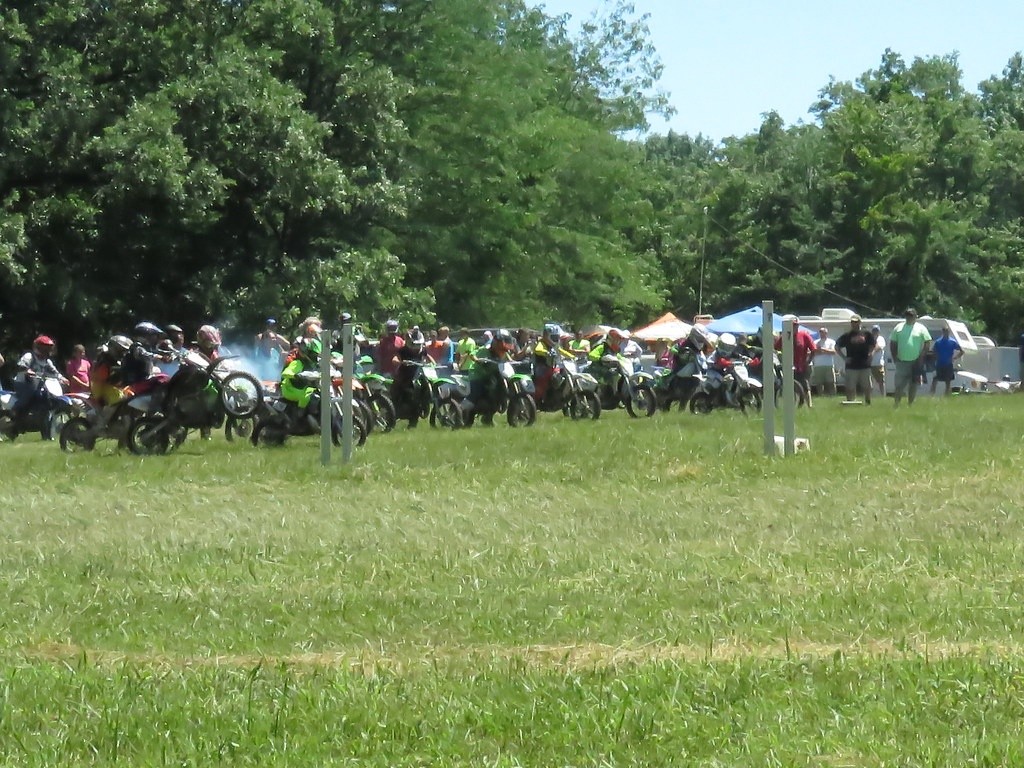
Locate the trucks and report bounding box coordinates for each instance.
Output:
[799,308,1021,398]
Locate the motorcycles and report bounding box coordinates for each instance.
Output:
[0,336,807,455]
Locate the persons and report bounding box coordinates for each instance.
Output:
[1018,324,1024,389]
[0,323,222,452]
[870,325,886,397]
[929,327,965,396]
[649,324,784,410]
[774,319,818,407]
[255,312,371,427]
[812,328,837,396]
[889,308,931,406]
[834,315,876,405]
[377,319,642,429]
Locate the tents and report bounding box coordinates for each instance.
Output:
[707,306,818,339]
[630,313,716,342]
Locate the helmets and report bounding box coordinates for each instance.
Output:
[36,311,799,375]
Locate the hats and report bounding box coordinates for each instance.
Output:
[872,325,880,330]
[905,308,917,317]
[850,314,861,322]
